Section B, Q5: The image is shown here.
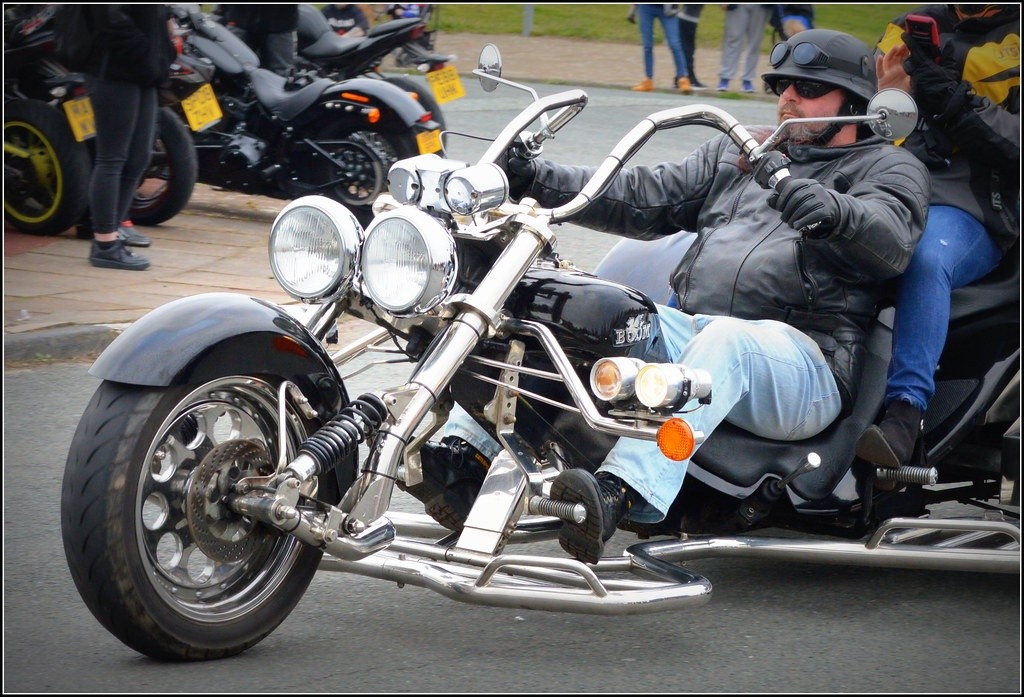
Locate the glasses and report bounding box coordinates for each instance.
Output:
[768,41,831,70]
[775,77,840,99]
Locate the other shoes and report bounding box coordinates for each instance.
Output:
[632,79,653,91]
[678,77,692,93]
[717,81,726,90]
[741,81,753,92]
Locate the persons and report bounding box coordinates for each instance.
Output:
[385,4,419,20]
[627,4,708,92]
[717,4,814,94]
[218,4,300,77]
[852,5,1020,491]
[56,5,176,270]
[322,4,368,36]
[395,29,930,565]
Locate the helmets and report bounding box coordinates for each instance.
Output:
[761,30,878,101]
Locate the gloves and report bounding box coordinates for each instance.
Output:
[766,177,839,233]
[901,32,960,114]
[498,146,537,202]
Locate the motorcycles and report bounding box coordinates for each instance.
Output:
[56,41,1021,664]
[3,2,467,241]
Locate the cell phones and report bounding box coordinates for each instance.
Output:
[905,15,942,66]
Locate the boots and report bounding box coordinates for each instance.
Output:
[550,468,633,564]
[856,401,920,465]
[363,420,490,532]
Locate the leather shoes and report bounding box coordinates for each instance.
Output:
[118,227,150,246]
[90,241,149,269]
